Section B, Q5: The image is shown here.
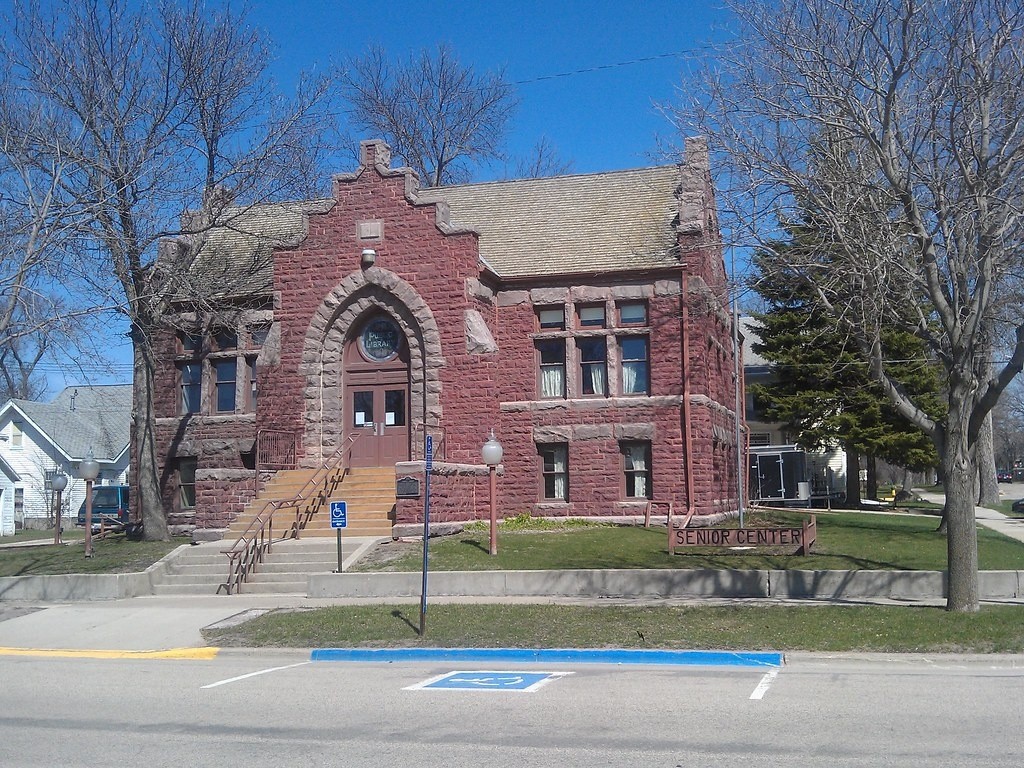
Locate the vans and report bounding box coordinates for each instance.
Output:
[74,485,129,536]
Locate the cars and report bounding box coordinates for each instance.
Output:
[997,473,1012,484]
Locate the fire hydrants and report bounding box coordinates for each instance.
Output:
[891,487,895,496]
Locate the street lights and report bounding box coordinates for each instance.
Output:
[481,427,503,555]
[52,468,68,545]
[80,446,99,558]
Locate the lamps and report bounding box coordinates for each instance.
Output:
[361,249,376,263]
